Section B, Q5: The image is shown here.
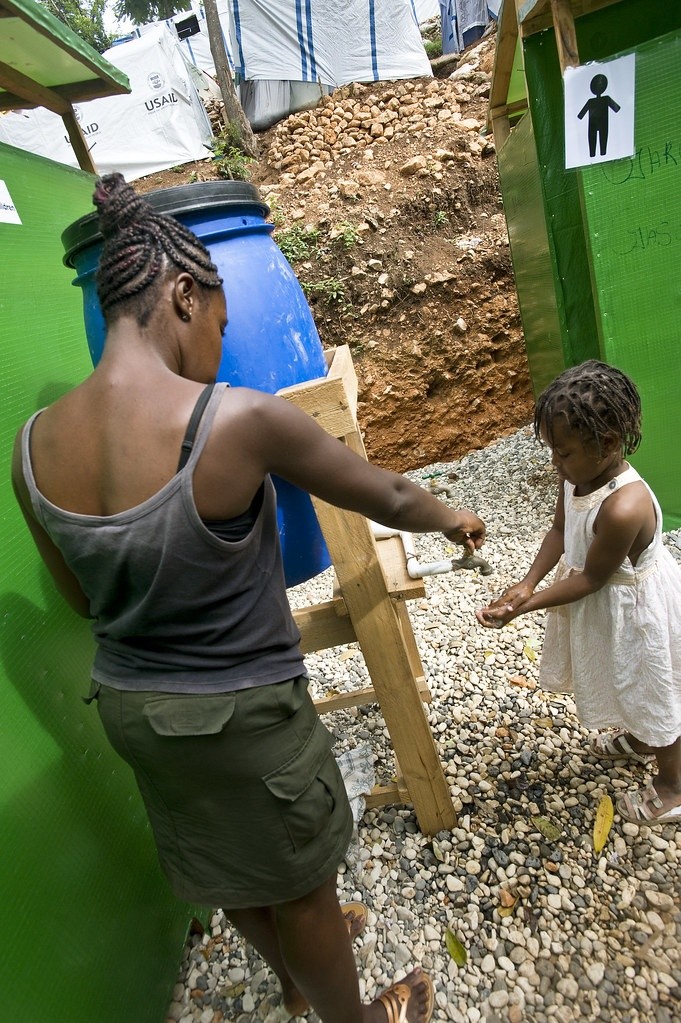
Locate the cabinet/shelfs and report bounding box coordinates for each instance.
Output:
[263,342,457,834]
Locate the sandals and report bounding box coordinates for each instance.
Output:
[592,728,657,770]
[615,776,681,824]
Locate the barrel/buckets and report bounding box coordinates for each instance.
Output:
[59,181,346,590]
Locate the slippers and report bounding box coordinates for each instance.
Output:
[287,900,367,1017]
[370,972,434,1023]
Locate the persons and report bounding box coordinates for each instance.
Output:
[10,170,487,1023]
[473,358,681,824]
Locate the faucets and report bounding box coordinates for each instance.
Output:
[451,533,494,577]
